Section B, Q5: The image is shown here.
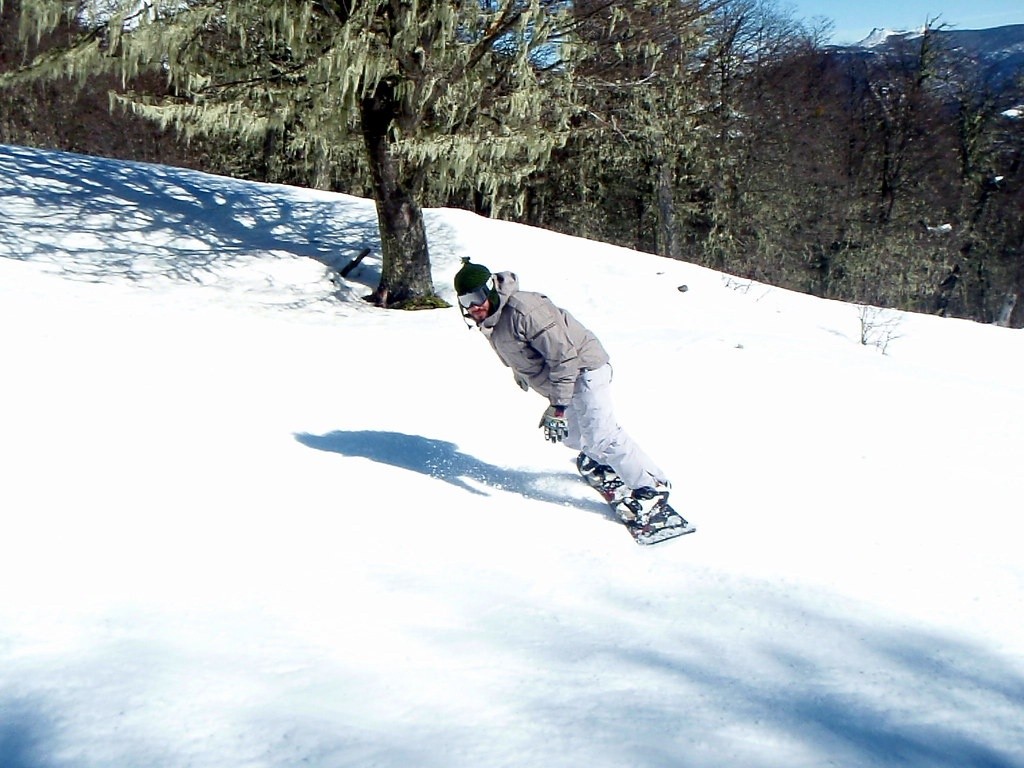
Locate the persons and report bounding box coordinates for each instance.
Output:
[454,257,672,529]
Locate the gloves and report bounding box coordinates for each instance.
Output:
[538,405,569,443]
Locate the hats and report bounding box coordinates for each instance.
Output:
[454,256,500,330]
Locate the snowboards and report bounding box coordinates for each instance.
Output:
[575,451,699,546]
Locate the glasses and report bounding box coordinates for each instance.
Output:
[457,275,494,311]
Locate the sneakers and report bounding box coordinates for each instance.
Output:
[615,479,672,527]
[588,464,624,490]
